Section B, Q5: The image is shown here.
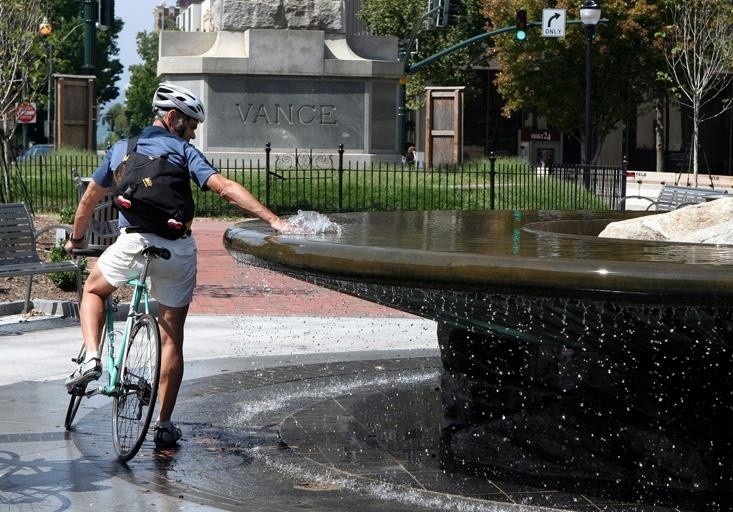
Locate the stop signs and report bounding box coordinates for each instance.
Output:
[14,104,36,123]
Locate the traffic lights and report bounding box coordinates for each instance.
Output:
[435,0,462,30]
[515,8,528,42]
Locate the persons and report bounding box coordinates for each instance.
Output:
[405,143,417,166]
[61,84,319,447]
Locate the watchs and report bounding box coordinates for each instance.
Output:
[68,231,87,245]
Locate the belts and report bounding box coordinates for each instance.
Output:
[125,227,143,234]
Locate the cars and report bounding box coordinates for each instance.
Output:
[13,143,57,164]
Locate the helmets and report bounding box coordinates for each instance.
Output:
[152,84,205,123]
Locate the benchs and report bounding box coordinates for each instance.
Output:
[618,185,728,216]
[0,174,120,313]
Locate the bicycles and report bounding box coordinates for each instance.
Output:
[58,238,172,463]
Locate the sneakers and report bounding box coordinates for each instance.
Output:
[63,357,103,386]
[154,421,182,446]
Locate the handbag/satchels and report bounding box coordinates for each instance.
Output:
[112,152,196,242]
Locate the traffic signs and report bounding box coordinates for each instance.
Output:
[541,9,565,37]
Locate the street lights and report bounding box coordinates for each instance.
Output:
[578,0,602,195]
[46,14,111,145]
